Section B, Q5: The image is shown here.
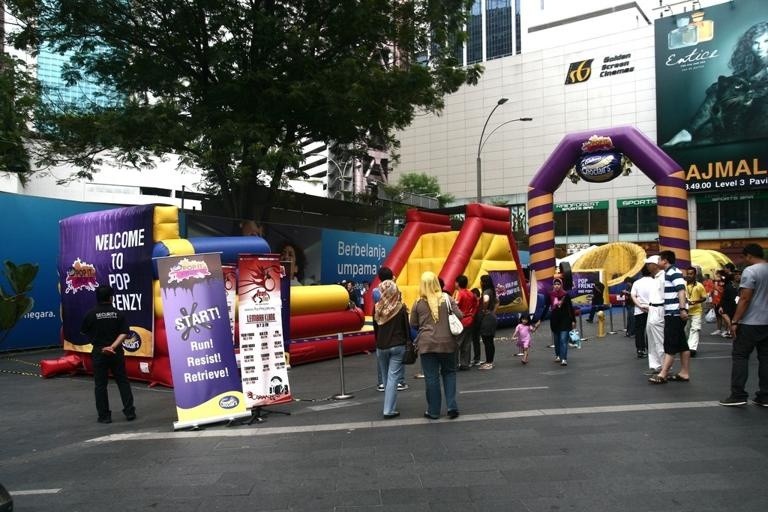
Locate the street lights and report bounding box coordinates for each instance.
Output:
[477,98,533,203]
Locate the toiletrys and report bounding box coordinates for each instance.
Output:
[688,11,714,44]
[666,16,698,51]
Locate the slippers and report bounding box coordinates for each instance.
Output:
[648,374,667,383]
[668,374,690,381]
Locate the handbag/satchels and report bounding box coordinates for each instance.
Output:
[443,292,463,335]
[402,304,416,364]
[569,327,581,349]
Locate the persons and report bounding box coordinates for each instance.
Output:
[274,237,306,287]
[586,278,606,323]
[685,266,708,360]
[334,280,370,309]
[372,266,410,391]
[631,262,654,358]
[662,21,768,151]
[371,279,410,418]
[479,274,499,371]
[452,275,477,372]
[232,219,263,238]
[512,313,536,365]
[719,242,768,408]
[80,284,138,424]
[535,278,577,365]
[701,263,742,338]
[410,271,464,420]
[642,255,666,375]
[620,276,635,339]
[648,250,690,384]
[470,287,482,368]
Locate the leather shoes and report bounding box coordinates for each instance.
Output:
[128,415,135,420]
[97,417,111,423]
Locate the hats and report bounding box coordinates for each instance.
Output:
[97,346,114,369]
[644,254,661,264]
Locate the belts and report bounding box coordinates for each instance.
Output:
[649,303,665,306]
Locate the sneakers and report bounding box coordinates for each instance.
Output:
[478,363,492,370]
[561,360,567,365]
[719,398,747,405]
[710,329,721,335]
[644,368,658,375]
[448,411,460,416]
[480,361,495,367]
[397,383,409,391]
[722,330,727,334]
[752,398,768,407]
[722,331,733,338]
[376,384,385,391]
[424,410,439,419]
[553,356,560,361]
